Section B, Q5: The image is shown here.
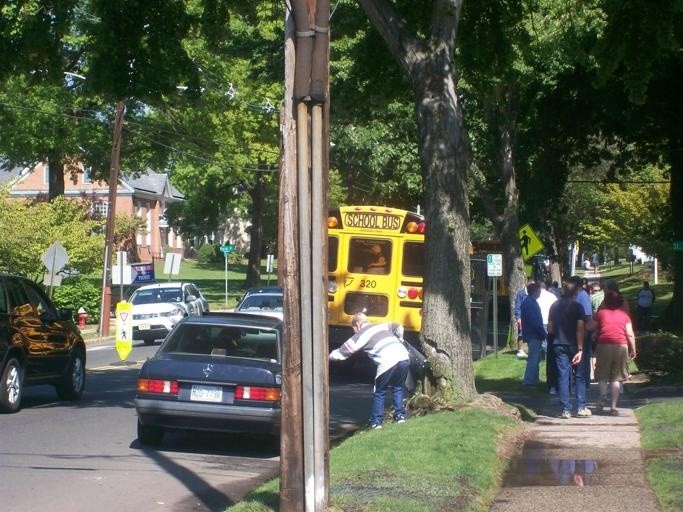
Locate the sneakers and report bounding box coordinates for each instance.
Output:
[515,349,528,358]
[397,416,406,423]
[371,424,382,430]
[518,382,621,418]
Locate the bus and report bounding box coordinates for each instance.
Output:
[327,206,426,377]
[470,238,511,321]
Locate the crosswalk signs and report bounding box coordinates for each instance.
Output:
[516,223,546,262]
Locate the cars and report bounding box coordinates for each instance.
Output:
[530,254,551,278]
[0,273,86,413]
[127,281,283,446]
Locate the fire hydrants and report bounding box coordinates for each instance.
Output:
[76,307,90,328]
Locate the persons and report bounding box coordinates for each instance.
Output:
[516,445,600,487]
[329,311,411,429]
[367,244,388,268]
[635,282,656,334]
[582,258,591,273]
[590,251,600,275]
[514,273,638,420]
